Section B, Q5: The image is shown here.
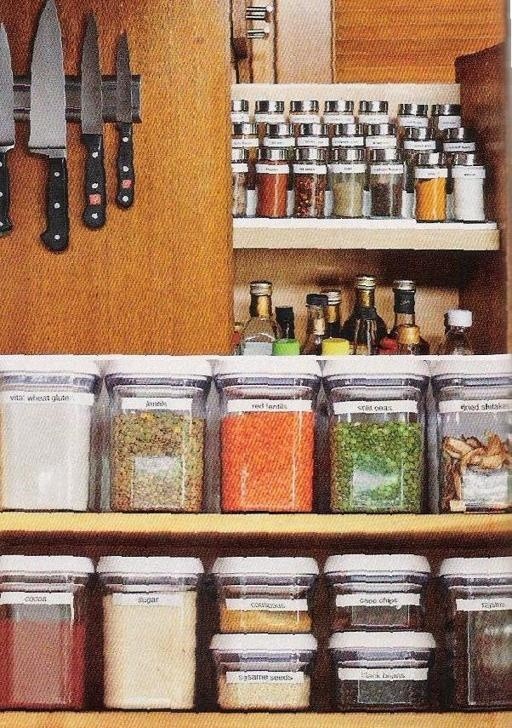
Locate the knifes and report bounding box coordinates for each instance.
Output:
[114,28,135,210]
[78,7,108,231]
[0,21,17,233]
[26,0,70,251]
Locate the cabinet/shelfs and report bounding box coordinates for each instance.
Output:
[0,356,511,728]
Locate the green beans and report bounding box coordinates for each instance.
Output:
[109,412,207,511]
[328,421,425,514]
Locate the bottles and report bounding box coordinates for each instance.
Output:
[437,555,512,711]
[328,145,367,218]
[253,148,291,218]
[293,147,328,219]
[99,355,214,512]
[240,274,476,354]
[451,152,486,222]
[2,353,103,512]
[231,99,476,151]
[430,354,512,513]
[369,147,405,219]
[0,551,204,713]
[322,354,431,514]
[212,356,321,514]
[232,146,249,218]
[413,152,448,221]
